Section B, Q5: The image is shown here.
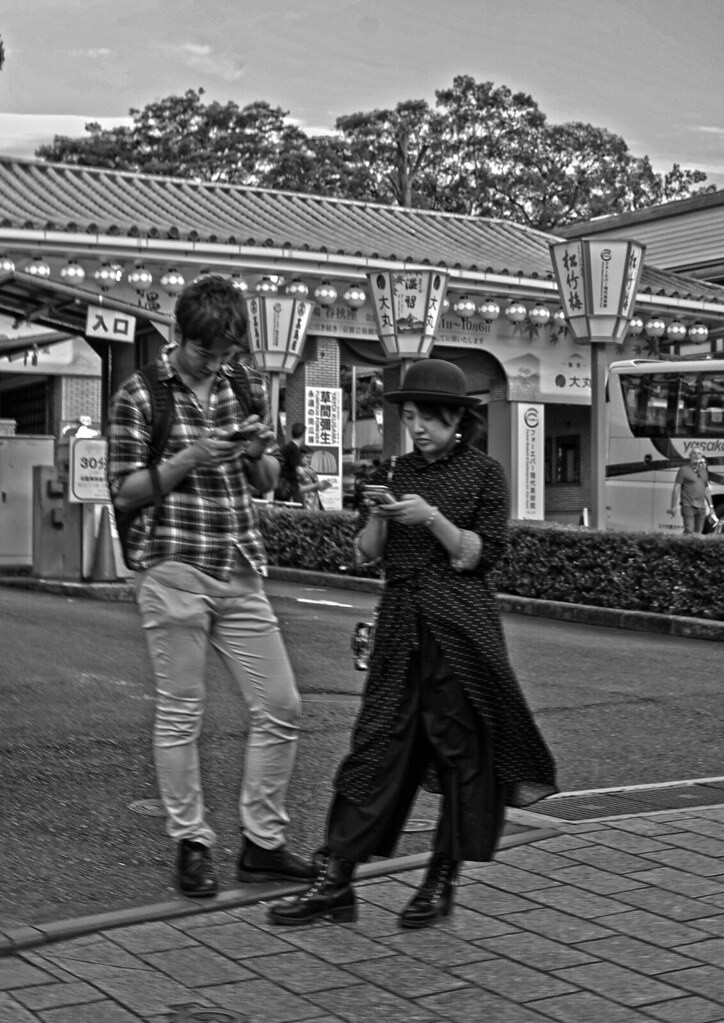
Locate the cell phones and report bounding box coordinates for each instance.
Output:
[220,426,260,442]
[361,484,399,506]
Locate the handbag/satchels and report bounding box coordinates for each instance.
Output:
[350,621,376,672]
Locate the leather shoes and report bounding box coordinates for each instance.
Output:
[236,838,323,882]
[176,839,219,895]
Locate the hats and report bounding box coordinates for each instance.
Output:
[382,359,481,404]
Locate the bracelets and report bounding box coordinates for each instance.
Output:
[424,506,439,528]
[709,505,714,508]
[242,453,264,463]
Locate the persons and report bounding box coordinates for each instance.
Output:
[268,359,560,928]
[106,277,313,898]
[669,446,716,534]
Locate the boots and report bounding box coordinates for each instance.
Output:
[398,850,458,927]
[265,850,356,922]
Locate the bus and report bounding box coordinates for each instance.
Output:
[604,358,723,541]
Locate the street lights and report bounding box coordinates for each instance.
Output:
[549,236,653,530]
[244,291,315,508]
[365,266,452,474]
[82,506,126,586]
